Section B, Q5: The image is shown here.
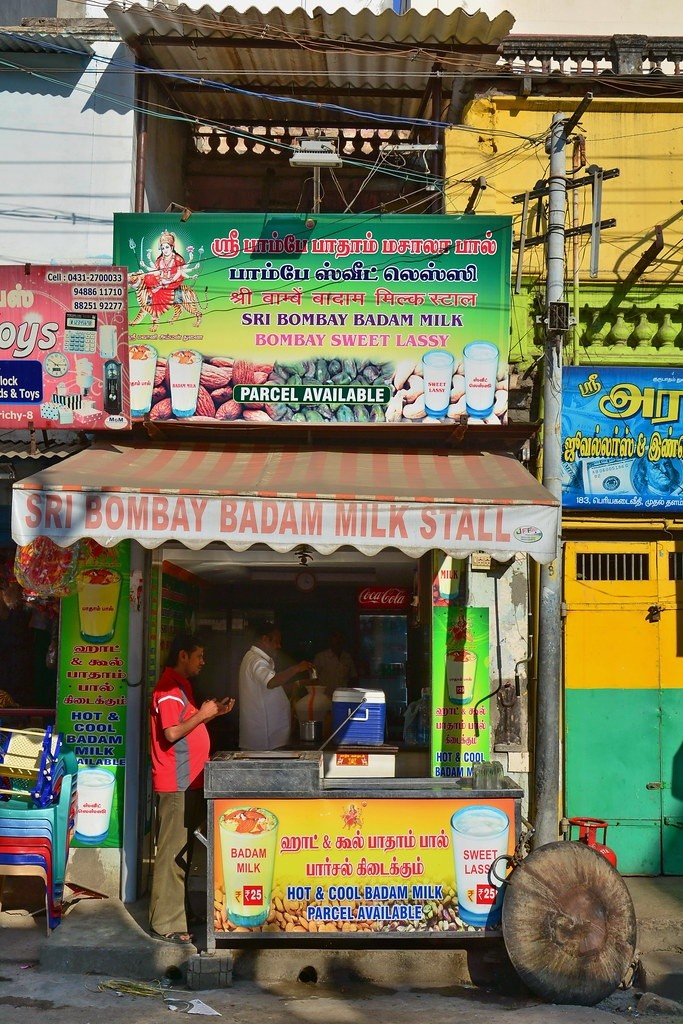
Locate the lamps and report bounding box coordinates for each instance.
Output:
[289,137,343,168]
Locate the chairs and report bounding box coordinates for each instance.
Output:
[0,725,79,937]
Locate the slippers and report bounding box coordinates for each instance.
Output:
[151,929,196,943]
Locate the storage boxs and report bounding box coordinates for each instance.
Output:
[332,688,386,746]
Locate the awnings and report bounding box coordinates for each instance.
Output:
[10,438,559,567]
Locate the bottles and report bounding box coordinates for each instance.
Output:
[417,687,430,744]
[295,685,334,738]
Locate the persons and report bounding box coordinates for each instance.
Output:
[148,634,236,944]
[240,622,356,751]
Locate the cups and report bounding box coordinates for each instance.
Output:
[128,344,158,417]
[218,806,279,927]
[462,340,500,418]
[75,567,122,644]
[168,348,202,417]
[73,766,116,845]
[99,324,117,359]
[450,805,510,928]
[422,350,455,417]
[446,650,478,705]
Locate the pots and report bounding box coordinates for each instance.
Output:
[299,720,323,741]
